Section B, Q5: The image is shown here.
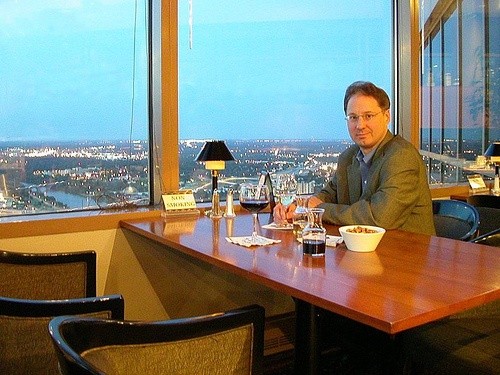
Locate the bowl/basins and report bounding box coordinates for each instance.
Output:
[339,226,386,252]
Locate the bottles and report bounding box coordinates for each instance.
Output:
[302,209,326,256]
[293,198,309,238]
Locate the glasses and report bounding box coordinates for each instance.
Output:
[344,108,387,121]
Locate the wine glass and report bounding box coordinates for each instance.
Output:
[239,185,270,243]
[277,175,297,227]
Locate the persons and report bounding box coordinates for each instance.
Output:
[285,81,437,237]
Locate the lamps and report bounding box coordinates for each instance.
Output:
[193,141,237,218]
[485,143,500,178]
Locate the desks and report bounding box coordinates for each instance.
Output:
[119,210,500,375]
[463,160,500,179]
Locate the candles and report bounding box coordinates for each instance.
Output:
[226,191,233,215]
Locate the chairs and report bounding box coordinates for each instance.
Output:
[432,200,500,247]
[1,249,265,375]
